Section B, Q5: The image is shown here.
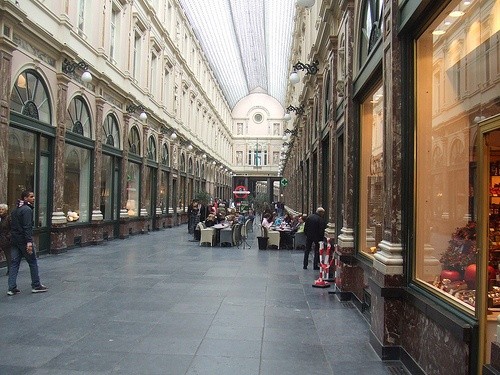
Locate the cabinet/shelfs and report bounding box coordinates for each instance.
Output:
[468,160,500,252]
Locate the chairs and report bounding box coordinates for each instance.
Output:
[195,220,217,247]
[258,223,280,250]
[293,232,307,251]
[219,219,254,248]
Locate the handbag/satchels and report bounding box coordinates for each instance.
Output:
[205,219,214,227]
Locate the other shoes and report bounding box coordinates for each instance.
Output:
[313,267,320,270]
[6,271,9,276]
[303,265,307,270]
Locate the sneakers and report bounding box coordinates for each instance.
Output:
[31,283,49,292]
[7,288,21,296]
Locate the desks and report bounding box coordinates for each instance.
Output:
[211,223,231,247]
[273,228,295,251]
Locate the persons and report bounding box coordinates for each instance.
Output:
[187,198,254,234]
[0,204,10,276]
[303,207,326,270]
[262,201,284,230]
[7,190,48,296]
[280,214,305,250]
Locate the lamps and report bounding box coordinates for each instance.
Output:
[62,58,93,84]
[279,141,294,152]
[288,59,319,85]
[161,124,178,140]
[195,149,234,176]
[282,127,298,141]
[126,104,147,121]
[283,104,305,121]
[277,152,291,176]
[473,107,494,124]
[180,137,193,152]
[296,0,316,9]
